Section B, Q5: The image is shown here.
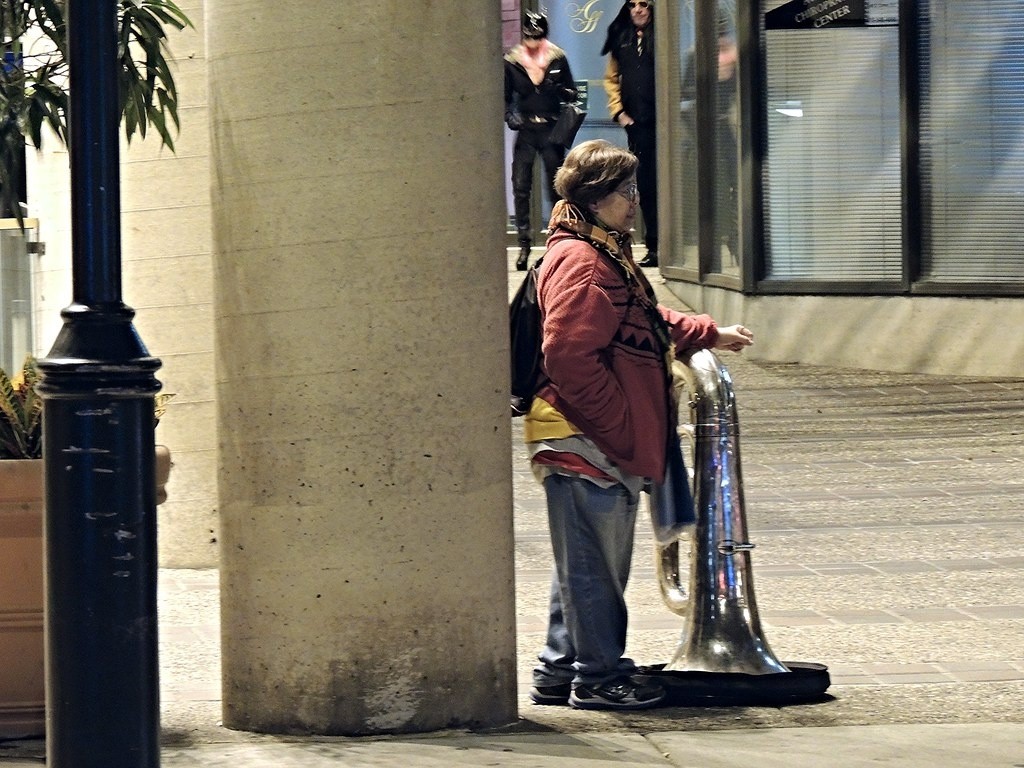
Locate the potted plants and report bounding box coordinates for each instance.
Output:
[0,352,177,743]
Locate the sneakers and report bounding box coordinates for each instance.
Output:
[569,675,669,709]
[529,682,572,704]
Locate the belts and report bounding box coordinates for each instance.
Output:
[529,115,559,124]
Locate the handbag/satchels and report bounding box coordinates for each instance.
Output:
[547,88,588,149]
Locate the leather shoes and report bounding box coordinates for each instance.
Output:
[638,251,658,267]
[516,241,531,270]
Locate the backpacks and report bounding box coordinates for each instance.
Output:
[510,236,635,418]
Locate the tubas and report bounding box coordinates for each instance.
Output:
[625,344,832,704]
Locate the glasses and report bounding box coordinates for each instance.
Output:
[614,185,641,206]
[521,30,542,40]
[626,1,652,9]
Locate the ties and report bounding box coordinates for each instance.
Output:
[637,31,644,57]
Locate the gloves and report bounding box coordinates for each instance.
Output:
[507,113,526,130]
[549,83,569,102]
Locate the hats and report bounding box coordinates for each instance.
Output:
[523,12,548,33]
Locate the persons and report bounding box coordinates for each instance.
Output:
[681,10,739,264]
[530,140,755,707]
[504,12,578,270]
[602,0,658,266]
